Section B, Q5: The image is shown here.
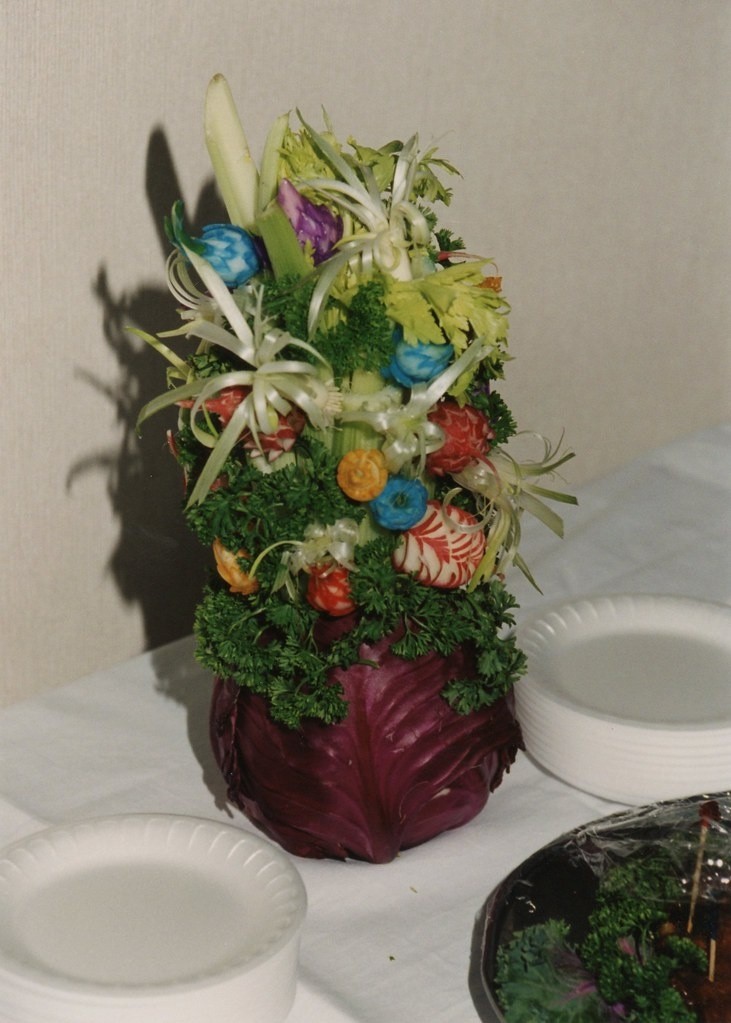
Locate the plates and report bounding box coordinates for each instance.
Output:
[514,593,731,805]
[469,791,731,1023]
[0,814,308,1023]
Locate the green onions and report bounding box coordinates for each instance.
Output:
[124,114,580,592]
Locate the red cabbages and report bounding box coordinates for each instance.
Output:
[210,602,528,864]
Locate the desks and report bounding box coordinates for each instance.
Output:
[1,419,731,1023]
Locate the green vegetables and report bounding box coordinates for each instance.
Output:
[123,105,526,726]
[493,817,730,1023]
